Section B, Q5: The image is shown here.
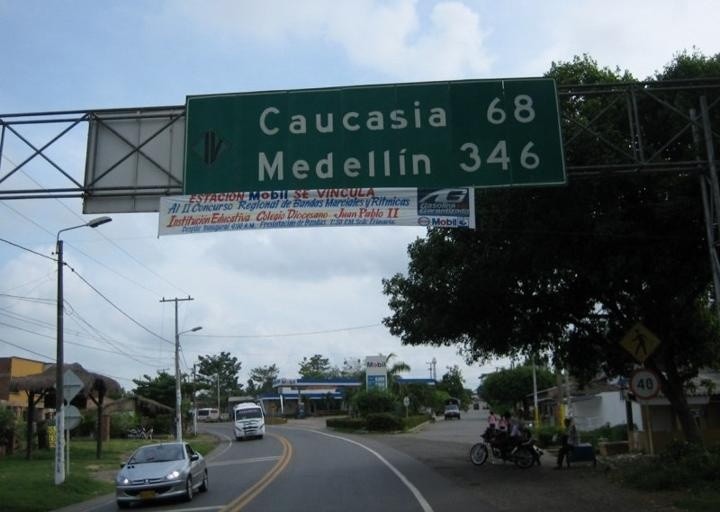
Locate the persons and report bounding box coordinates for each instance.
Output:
[553,419,576,470]
[488,409,524,458]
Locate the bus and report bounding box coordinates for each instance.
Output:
[231,400,267,441]
[197,408,219,422]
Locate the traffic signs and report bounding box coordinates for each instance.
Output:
[181,76,566,201]
[628,367,662,402]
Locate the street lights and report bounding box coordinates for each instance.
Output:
[175,325,204,441]
[51,215,115,485]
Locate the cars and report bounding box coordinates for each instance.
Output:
[472,403,480,411]
[443,404,462,420]
[113,441,211,509]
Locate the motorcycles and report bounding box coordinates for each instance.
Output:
[467,423,542,469]
